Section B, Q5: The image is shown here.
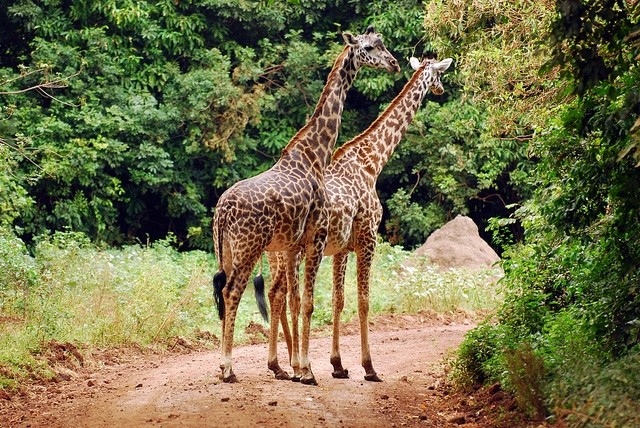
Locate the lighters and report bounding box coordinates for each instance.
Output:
[211,24,401,386]
[252,55,453,383]
